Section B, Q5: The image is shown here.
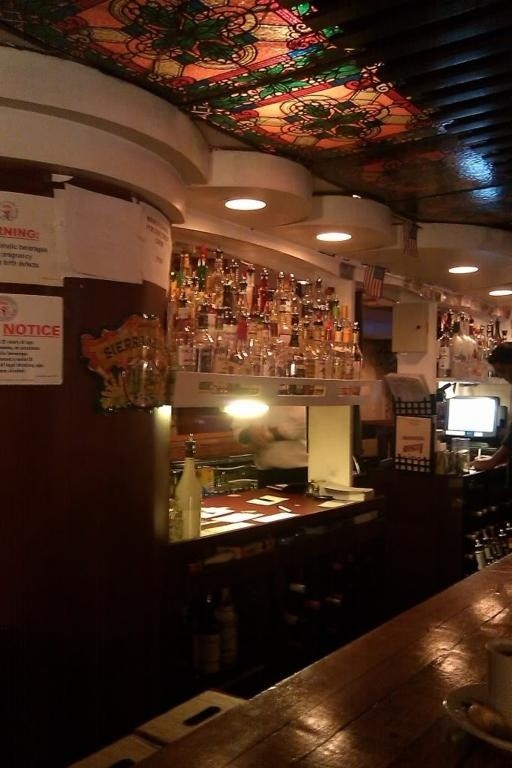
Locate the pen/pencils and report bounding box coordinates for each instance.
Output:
[478,446,482,459]
[259,498,273,501]
[278,505,292,512]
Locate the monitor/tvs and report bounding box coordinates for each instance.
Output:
[444,396,500,438]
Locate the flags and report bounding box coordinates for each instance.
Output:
[361,263,387,299]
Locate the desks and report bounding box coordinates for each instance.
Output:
[130,552,512,768]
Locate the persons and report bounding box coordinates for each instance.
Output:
[473,340,511,475]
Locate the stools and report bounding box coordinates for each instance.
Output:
[64,688,251,768]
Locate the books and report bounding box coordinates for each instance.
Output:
[311,478,376,503]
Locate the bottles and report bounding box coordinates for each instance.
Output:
[188,589,239,679]
[174,439,203,542]
[471,520,512,570]
[450,436,471,475]
[437,310,502,380]
[170,243,364,396]
